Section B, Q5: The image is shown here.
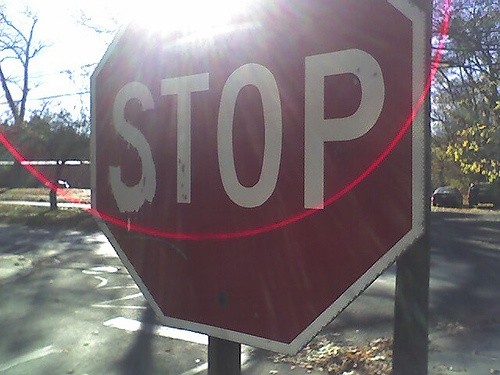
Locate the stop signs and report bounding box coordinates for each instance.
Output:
[89,0,430,357]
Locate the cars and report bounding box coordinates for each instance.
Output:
[467,180,500,208]
[432,186,464,208]
